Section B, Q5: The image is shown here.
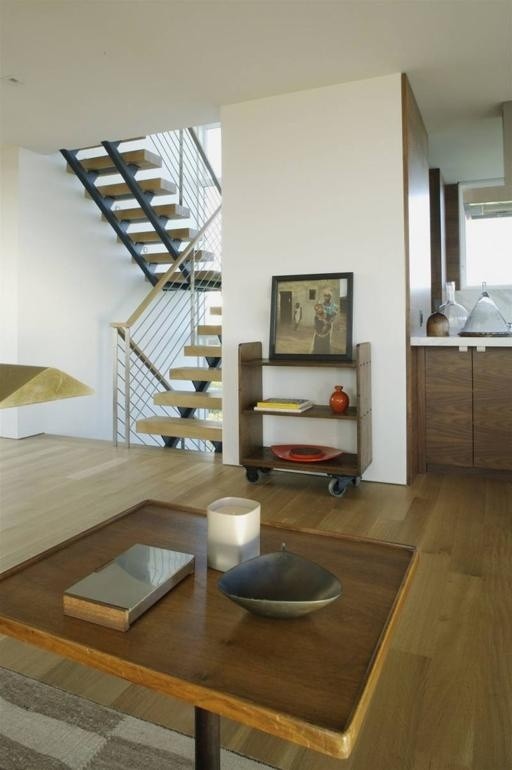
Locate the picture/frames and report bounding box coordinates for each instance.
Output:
[267,270,355,363]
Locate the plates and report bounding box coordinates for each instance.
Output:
[270,444,345,462]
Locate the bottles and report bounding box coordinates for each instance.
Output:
[329,385,350,415]
[438,281,470,336]
[461,282,510,333]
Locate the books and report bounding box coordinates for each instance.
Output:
[253,397,313,414]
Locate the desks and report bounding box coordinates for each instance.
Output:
[0,495,423,770]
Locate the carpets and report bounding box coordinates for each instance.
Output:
[0,663,285,770]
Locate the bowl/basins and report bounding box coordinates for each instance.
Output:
[216,549,343,620]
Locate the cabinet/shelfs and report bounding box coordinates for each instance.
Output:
[473,347,511,470]
[423,346,473,469]
[233,340,372,499]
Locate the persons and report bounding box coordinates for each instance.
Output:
[310,304,332,353]
[292,303,302,330]
[311,288,338,351]
